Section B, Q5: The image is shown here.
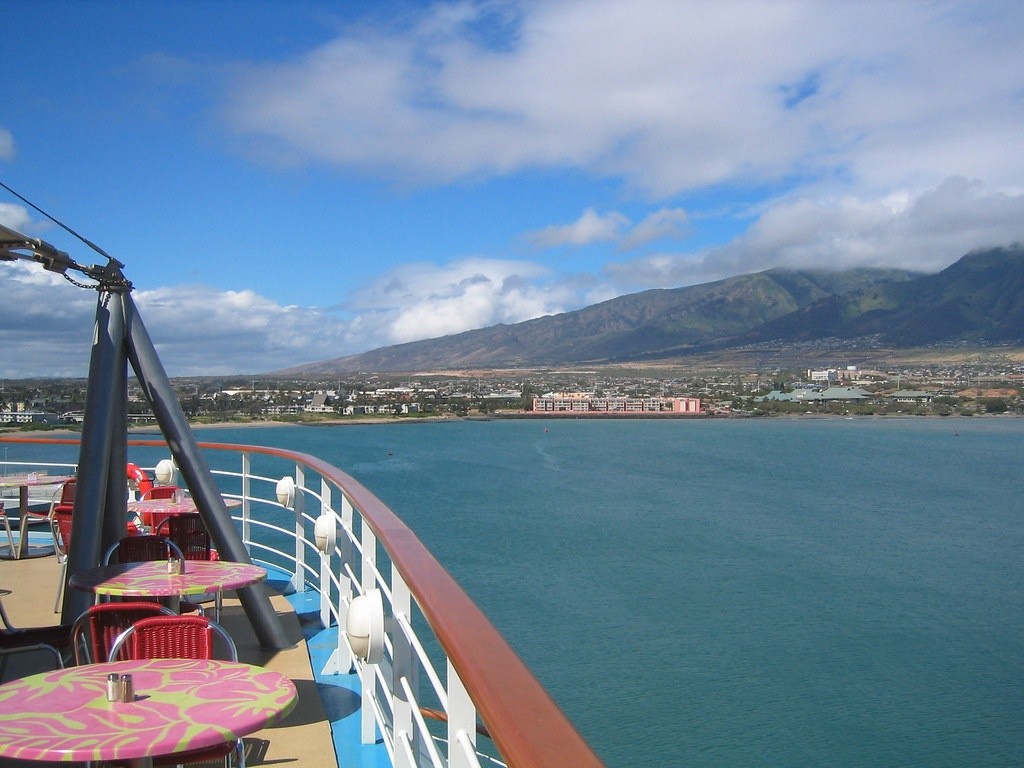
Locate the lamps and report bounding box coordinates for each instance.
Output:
[274,475,298,510]
[312,513,339,556]
[344,597,383,665]
[153,457,179,487]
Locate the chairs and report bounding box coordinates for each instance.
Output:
[103,539,205,618]
[106,615,248,768]
[0,475,77,564]
[0,586,92,684]
[159,512,223,623]
[71,599,181,667]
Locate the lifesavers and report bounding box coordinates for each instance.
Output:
[128,462,154,535]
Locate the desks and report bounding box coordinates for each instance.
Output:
[66,551,270,625]
[0,656,300,768]
[0,475,70,561]
[128,495,245,525]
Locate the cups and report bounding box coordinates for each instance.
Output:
[175,490,184,504]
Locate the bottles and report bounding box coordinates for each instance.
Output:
[178,558,185,575]
[167,557,175,574]
[106,674,121,702]
[121,674,134,703]
